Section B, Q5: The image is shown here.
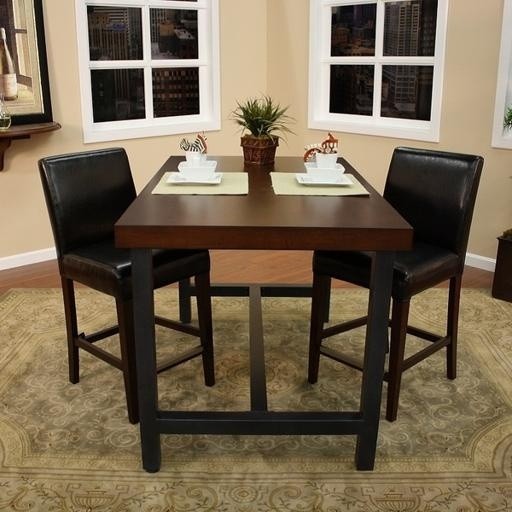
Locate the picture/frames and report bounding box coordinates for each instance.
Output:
[0,0,53,125]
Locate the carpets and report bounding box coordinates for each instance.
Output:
[0,287,512,511]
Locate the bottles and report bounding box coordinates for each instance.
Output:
[0,28,18,100]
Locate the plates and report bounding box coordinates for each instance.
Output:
[167,174,223,184]
[296,173,351,185]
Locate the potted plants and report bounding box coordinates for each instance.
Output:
[230,92,297,167]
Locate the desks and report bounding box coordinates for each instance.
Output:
[114,157,414,473]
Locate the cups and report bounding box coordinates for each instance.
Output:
[316,153,337,167]
[185,152,199,166]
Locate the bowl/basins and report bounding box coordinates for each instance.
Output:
[305,163,346,180]
[178,160,217,178]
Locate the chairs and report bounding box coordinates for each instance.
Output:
[38,147,215,424]
[308,146,484,419]
